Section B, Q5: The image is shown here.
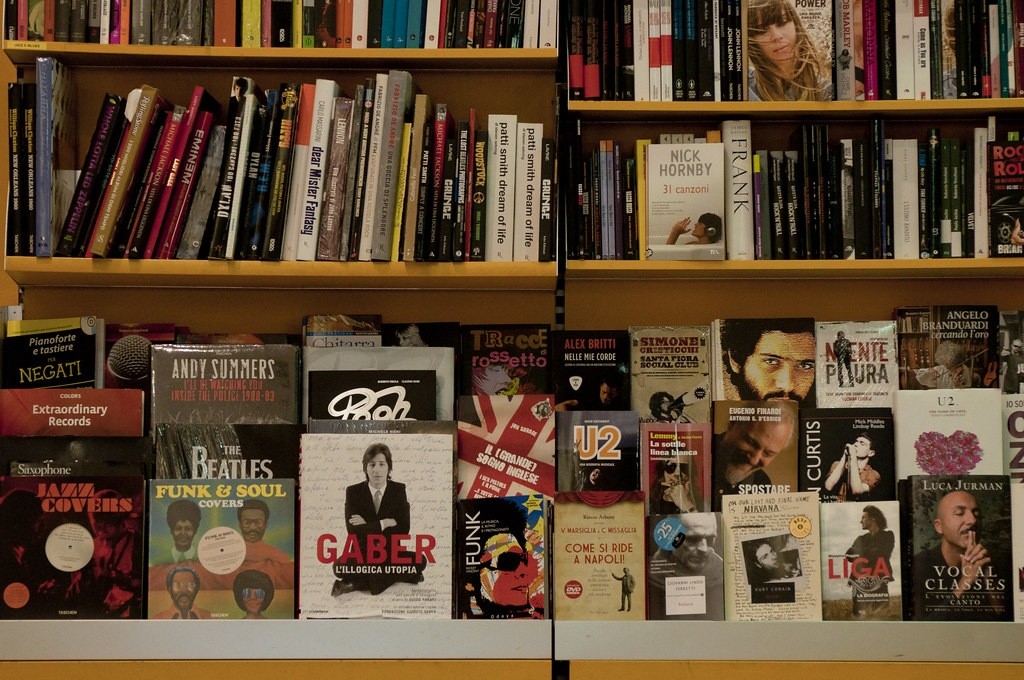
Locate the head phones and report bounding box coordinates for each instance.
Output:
[706,227,716,237]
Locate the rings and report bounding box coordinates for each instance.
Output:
[669,481,677,485]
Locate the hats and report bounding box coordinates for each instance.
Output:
[233,568,274,611]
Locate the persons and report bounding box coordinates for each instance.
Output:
[844,506,896,617]
[913,489,1012,619]
[649,511,723,619]
[231,77,248,106]
[610,567,637,612]
[826,432,880,501]
[61,490,142,618]
[1000,337,1024,394]
[652,446,705,514]
[556,381,626,411]
[834,330,856,386]
[330,443,427,597]
[0,489,59,621]
[746,0,835,104]
[394,324,428,347]
[711,402,794,511]
[917,341,973,389]
[0,317,1024,622]
[749,542,801,580]
[666,213,722,245]
[717,320,814,407]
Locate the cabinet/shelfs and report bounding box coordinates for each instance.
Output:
[564,97,1024,679]
[0,40,560,680]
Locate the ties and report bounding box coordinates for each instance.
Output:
[374,491,382,515]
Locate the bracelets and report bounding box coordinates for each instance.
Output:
[683,505,696,513]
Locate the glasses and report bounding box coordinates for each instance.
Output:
[477,552,529,571]
[172,582,196,592]
[242,519,264,528]
[664,460,690,476]
[1013,345,1021,348]
[241,588,265,600]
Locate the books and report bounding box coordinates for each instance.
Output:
[0,0,1024,262]
[0,306,1024,623]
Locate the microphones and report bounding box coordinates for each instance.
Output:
[107,334,152,388]
